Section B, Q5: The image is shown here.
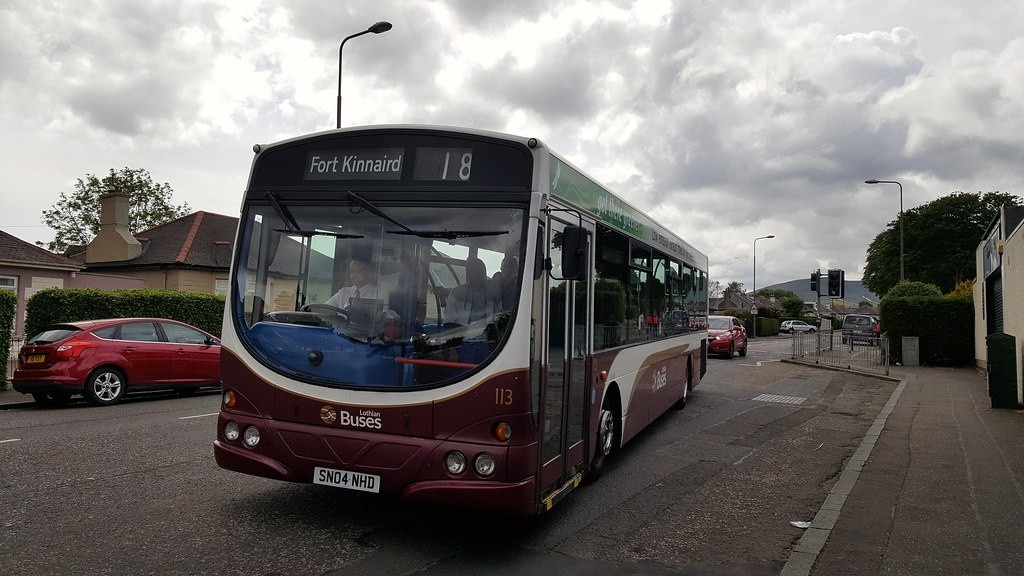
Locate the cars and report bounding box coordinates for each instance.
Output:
[13,318,221,406]
[709,316,747,359]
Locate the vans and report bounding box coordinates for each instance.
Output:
[842,315,876,346]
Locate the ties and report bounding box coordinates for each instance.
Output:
[355,289,361,298]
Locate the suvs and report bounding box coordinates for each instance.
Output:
[780,320,817,334]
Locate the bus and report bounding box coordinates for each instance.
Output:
[215,128,709,518]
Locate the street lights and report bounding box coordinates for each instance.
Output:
[337,21,393,128]
[753,236,775,337]
[865,180,904,282]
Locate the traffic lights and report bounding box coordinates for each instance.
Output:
[828,269,845,299]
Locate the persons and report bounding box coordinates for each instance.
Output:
[444,256,518,326]
[876,321,880,345]
[639,313,660,338]
[300,256,388,320]
